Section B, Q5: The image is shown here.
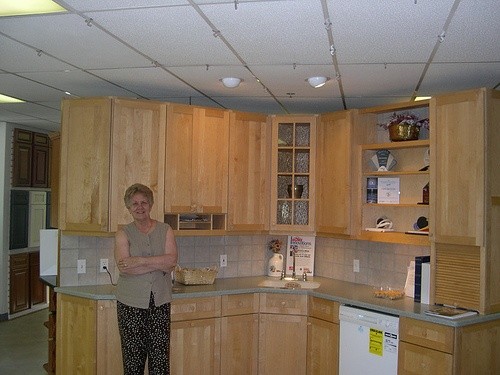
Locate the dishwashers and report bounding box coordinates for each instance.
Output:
[338,304,400,375]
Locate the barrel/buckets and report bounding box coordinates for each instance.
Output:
[287,184,303,199]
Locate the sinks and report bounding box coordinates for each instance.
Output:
[257,280,321,289]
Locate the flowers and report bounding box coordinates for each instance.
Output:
[269,239,283,253]
[378,110,429,132]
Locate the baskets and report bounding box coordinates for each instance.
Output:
[175,264,216,285]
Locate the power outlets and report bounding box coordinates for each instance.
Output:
[100,258,109,273]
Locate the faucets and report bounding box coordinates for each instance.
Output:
[281,270,284,279]
[303,272,306,282]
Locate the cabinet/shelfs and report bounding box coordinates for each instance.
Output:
[58,88,500,247]
[52,275,500,375]
[8,129,51,319]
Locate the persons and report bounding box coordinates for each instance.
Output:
[114,183,177,375]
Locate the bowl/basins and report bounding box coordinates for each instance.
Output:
[373,287,404,300]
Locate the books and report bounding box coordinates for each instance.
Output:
[424,308,479,320]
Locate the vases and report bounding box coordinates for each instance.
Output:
[388,124,420,141]
[268,254,284,277]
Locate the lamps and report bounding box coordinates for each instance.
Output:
[305,76,330,89]
[219,77,244,89]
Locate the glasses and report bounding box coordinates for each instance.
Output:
[128,200,149,207]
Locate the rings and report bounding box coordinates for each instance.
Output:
[125,264,127,267]
